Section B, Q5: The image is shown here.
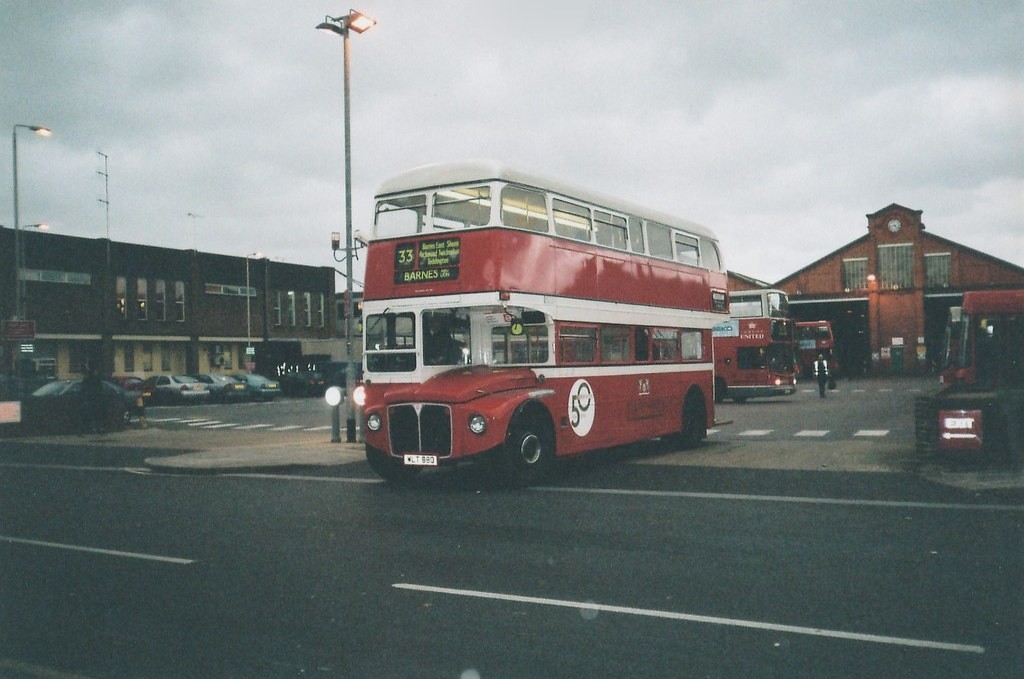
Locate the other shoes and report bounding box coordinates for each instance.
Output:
[819,393,826,398]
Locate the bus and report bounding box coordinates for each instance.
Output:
[794,319,837,380]
[710,288,798,403]
[316,161,731,485]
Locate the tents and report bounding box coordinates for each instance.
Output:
[961,287,1024,385]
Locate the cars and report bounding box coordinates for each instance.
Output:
[110,369,280,406]
[18,376,145,434]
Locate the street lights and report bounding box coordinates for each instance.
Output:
[246,252,260,373]
[315,8,378,443]
[10,122,52,412]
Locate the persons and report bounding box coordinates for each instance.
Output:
[812,352,830,398]
[424,313,460,366]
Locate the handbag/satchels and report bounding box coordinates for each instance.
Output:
[828,376,837,389]
